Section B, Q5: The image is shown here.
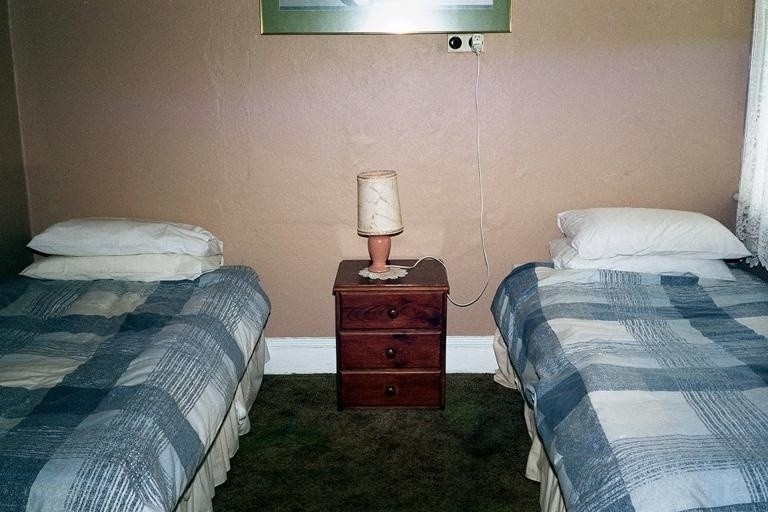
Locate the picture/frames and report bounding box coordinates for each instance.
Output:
[258,0,512,36]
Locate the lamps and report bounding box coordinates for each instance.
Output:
[355,169,410,281]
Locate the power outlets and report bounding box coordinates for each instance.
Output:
[445,34,485,54]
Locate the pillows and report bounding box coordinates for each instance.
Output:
[17,254,225,283]
[547,235,738,282]
[556,207,753,261]
[25,215,226,257]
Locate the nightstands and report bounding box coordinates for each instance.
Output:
[332,258,451,411]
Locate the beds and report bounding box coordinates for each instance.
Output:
[0,265,275,512]
[487,258,768,510]
